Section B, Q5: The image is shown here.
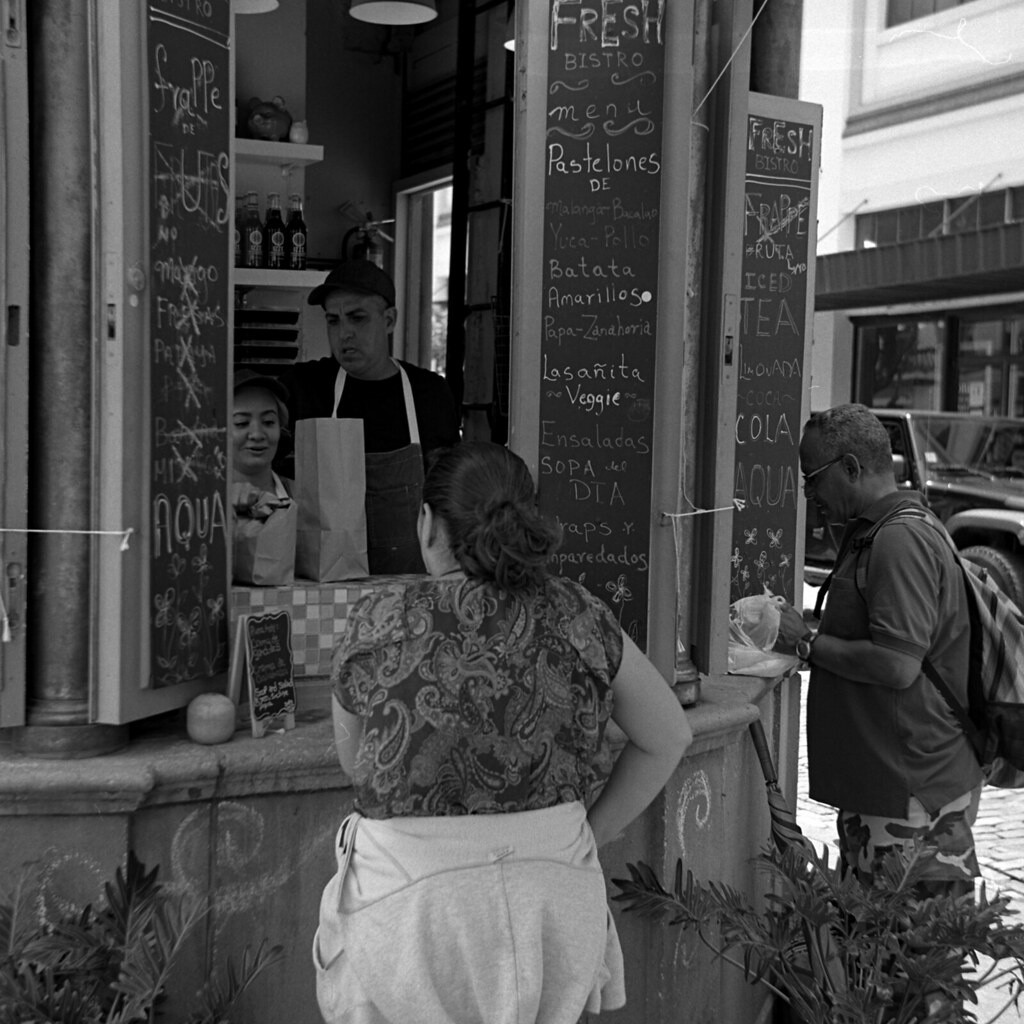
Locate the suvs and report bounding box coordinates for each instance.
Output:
[802,409,1024,615]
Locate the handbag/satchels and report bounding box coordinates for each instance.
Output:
[292,415,372,583]
[233,490,298,587]
[728,591,804,679]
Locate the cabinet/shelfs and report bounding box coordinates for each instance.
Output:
[235,139,330,290]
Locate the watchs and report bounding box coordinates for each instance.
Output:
[795,632,820,667]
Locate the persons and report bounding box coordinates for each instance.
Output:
[772,403,981,1024]
[311,442,693,1024]
[276,259,462,576]
[231,369,296,587]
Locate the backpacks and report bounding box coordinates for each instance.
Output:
[855,502,1024,794]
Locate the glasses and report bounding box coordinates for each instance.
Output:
[802,454,866,488]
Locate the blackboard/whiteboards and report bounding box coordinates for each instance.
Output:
[143,0,235,692]
[508,1,694,685]
[726,92,825,657]
[228,611,300,722]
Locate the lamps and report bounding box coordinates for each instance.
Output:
[350,0,438,24]
[503,9,515,51]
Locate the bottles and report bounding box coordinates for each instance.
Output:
[232,192,308,271]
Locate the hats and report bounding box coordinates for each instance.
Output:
[236,365,291,406]
[309,258,400,311]
